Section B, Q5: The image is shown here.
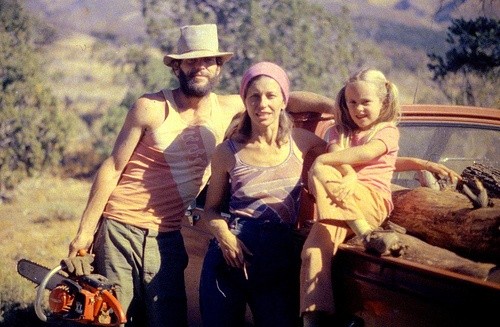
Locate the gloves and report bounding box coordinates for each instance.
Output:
[61,255,90,276]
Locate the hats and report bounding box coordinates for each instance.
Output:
[163,23,234,67]
[240,62,291,108]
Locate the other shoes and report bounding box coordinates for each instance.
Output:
[363,229,400,254]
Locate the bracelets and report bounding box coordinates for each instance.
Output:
[424,159,430,170]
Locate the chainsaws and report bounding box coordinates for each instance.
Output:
[16,249,127,327]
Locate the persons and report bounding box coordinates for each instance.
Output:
[299,68,403,326]
[198,62,464,327]
[67,22,337,326]
